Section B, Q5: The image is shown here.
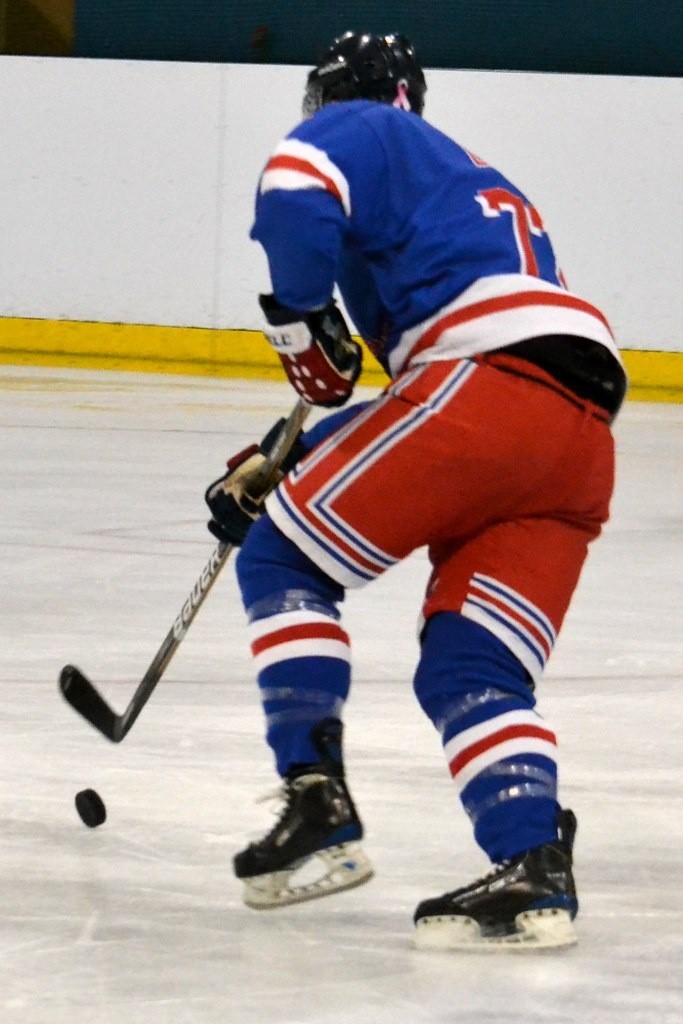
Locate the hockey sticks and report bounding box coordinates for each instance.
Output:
[56,308,344,744]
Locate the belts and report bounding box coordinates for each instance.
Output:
[467,357,612,425]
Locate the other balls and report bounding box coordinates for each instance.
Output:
[72,787,109,827]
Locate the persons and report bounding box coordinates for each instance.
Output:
[204,31,626,954]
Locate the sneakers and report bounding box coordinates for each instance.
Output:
[234,717,375,910]
[414,809,579,953]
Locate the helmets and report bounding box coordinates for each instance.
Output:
[302,29,427,120]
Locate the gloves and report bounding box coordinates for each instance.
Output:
[258,294,362,408]
[206,417,311,546]
